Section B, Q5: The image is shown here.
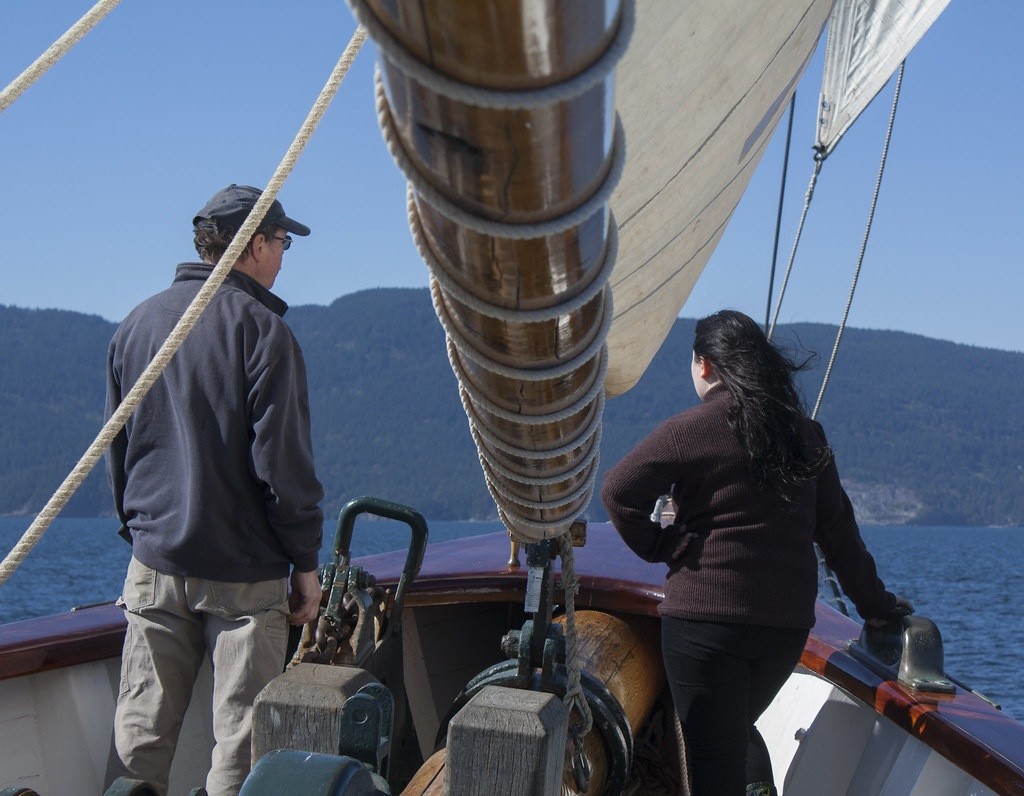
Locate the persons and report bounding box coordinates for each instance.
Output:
[597,309,917,796]
[101,183,329,796]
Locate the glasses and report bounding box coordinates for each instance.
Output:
[268,234,292,250]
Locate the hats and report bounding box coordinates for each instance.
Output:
[194,184,312,237]
